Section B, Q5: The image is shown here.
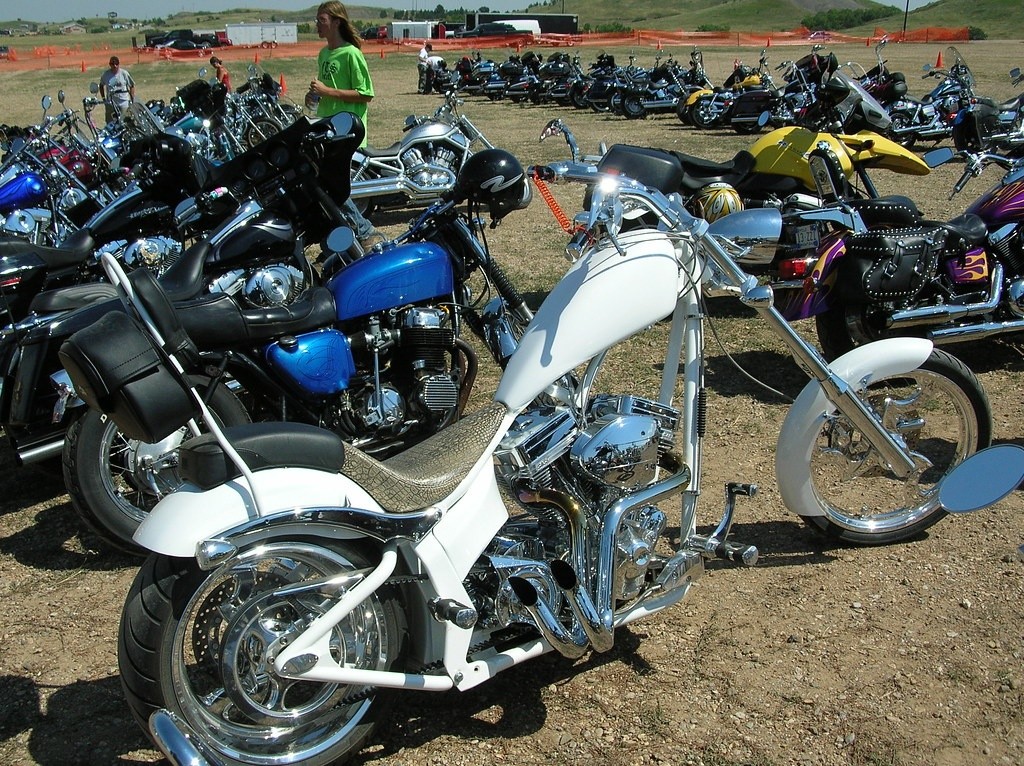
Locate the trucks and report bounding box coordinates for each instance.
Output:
[387,21,446,45]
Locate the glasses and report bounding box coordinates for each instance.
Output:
[313,16,327,24]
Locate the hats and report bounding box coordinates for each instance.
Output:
[426,44,434,51]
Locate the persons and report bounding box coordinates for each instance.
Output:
[305,0,375,147]
[210,57,233,95]
[99,56,136,124]
[417,44,447,95]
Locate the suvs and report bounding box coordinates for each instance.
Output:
[361,27,378,40]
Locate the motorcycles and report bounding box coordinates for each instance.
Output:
[794,40,889,133]
[670,59,754,126]
[529,49,930,287]
[431,52,640,112]
[686,51,780,129]
[722,44,838,133]
[783,147,1024,365]
[0,56,364,476]
[874,45,976,149]
[17,148,538,556]
[948,68,1024,159]
[345,68,534,219]
[616,47,716,119]
[54,143,997,766]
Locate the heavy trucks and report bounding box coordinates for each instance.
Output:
[215,22,299,49]
[445,12,582,47]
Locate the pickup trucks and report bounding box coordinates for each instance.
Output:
[151,28,215,50]
[456,23,532,47]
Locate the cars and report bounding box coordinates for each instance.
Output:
[378,26,387,38]
[154,39,206,59]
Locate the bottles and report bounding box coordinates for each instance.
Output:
[309,95,319,117]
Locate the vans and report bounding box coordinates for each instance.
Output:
[490,19,541,45]
[808,31,831,41]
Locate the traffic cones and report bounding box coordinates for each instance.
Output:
[279,72,287,91]
[516,41,522,52]
[656,40,662,50]
[866,37,871,47]
[935,51,944,68]
[766,37,772,48]
[379,47,385,59]
[254,52,259,62]
[80,60,86,71]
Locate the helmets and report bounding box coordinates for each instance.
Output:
[691,183,744,224]
[454,148,524,230]
